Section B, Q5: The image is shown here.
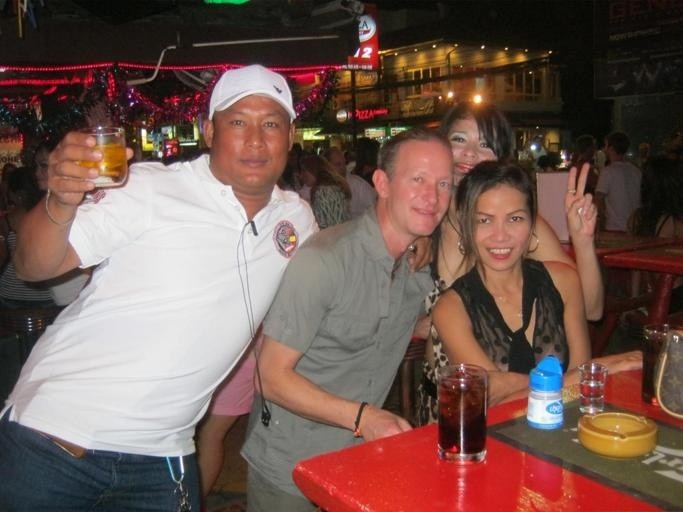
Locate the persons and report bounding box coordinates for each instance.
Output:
[1,124,682,501]
[0,64,437,511]
[413,101,607,421]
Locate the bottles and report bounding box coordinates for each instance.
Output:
[525,353,565,431]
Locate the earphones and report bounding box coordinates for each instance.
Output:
[262,412,268,426]
[264,404,271,419]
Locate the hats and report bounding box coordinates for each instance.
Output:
[208,64,298,124]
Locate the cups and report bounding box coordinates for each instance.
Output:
[578,359,606,414]
[639,323,671,406]
[436,361,489,464]
[76,125,130,188]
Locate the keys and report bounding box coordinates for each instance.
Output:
[177,498,192,512]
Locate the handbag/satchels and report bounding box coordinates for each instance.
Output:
[654,327,682,423]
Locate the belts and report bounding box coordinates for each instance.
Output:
[27,422,86,458]
